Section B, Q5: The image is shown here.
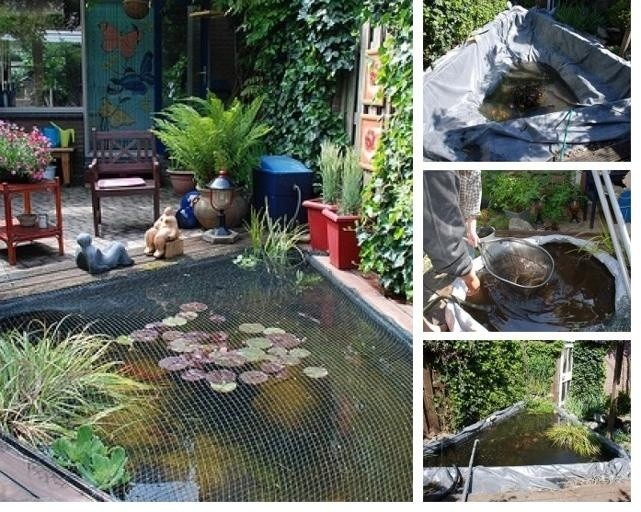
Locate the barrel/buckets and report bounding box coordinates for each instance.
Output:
[44,166,56,179]
[253,152,315,227]
[618,188,631,223]
[44,125,59,147]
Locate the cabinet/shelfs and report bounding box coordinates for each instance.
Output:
[0,177,64,266]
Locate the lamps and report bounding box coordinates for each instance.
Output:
[202,169,240,243]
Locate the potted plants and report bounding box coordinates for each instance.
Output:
[147,87,275,229]
[164,147,196,196]
[487,169,590,232]
[301,138,363,270]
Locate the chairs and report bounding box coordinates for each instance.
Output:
[84,127,160,237]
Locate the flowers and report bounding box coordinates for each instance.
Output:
[0,118,54,180]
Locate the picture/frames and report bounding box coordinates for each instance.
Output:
[362,50,385,107]
[358,114,383,172]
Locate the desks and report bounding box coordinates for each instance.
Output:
[50,147,75,184]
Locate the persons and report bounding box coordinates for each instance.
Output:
[423,170,480,297]
[457,170,482,247]
[144,207,177,258]
[75,234,135,275]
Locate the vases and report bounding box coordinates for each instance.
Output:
[0,167,42,184]
